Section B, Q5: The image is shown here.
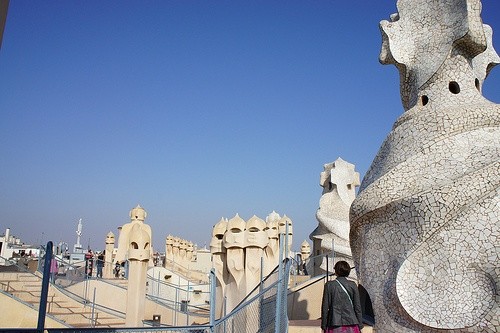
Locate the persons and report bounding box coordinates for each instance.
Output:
[303,263,308,275]
[20,253,28,270]
[115,261,120,277]
[50,256,58,284]
[85,250,93,276]
[321,261,364,333]
[97,254,104,278]
